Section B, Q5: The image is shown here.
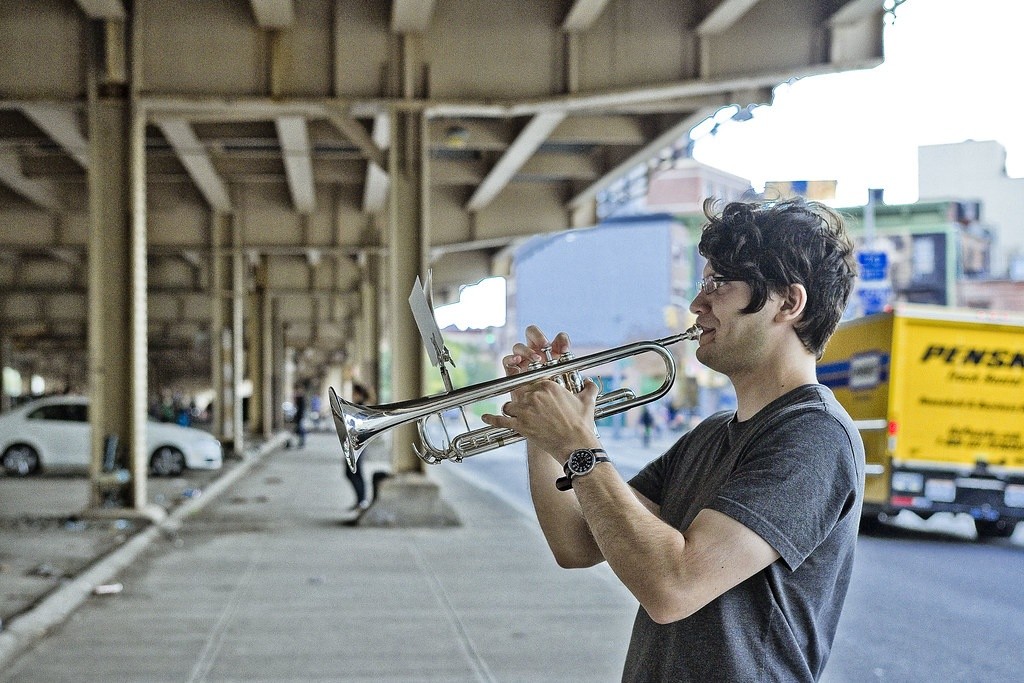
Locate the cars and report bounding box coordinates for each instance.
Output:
[0,394,224,480]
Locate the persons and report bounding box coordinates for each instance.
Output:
[342,384,392,526]
[483,196,866,683]
[293,389,307,448]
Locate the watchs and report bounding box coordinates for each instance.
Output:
[556,447,611,491]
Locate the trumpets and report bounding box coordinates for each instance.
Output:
[323,322,705,477]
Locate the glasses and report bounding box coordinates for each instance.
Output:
[698,273,753,295]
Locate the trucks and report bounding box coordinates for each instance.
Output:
[817,302,1024,539]
